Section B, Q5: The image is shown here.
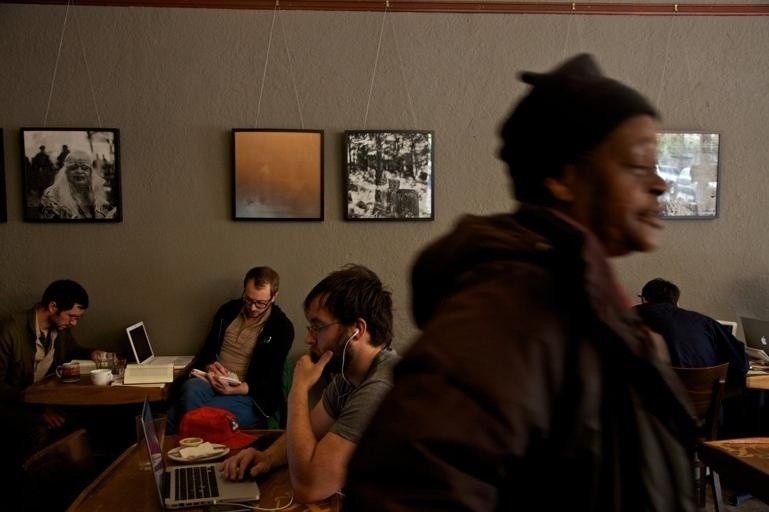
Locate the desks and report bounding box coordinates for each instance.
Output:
[22,353,196,411]
[64,428,345,510]
[746,354,769,414]
[700,432,769,507]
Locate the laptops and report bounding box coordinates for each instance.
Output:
[715,320,738,338]
[140,393,262,509]
[741,316,769,356]
[126,320,197,370]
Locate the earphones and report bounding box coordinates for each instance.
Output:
[351,327,360,338]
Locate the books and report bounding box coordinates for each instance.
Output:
[189,368,242,387]
[744,346,769,375]
[71,357,109,375]
[110,359,174,389]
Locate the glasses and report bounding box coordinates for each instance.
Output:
[240,289,275,308]
[306,323,340,335]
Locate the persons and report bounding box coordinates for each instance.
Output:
[343,48,707,512]
[1,279,104,510]
[217,260,403,507]
[166,265,294,435]
[634,278,748,438]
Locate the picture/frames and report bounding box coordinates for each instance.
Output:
[228,125,326,224]
[650,127,723,222]
[339,125,437,223]
[15,127,126,225]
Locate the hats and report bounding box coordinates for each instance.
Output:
[495,53,658,158]
[175,406,260,449]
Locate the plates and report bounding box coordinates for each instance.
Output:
[167,443,230,461]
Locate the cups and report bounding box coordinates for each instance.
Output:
[56,362,82,382]
[90,369,112,385]
[95,351,127,378]
[180,437,203,447]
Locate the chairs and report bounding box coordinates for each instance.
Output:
[673,360,732,509]
[21,428,88,493]
